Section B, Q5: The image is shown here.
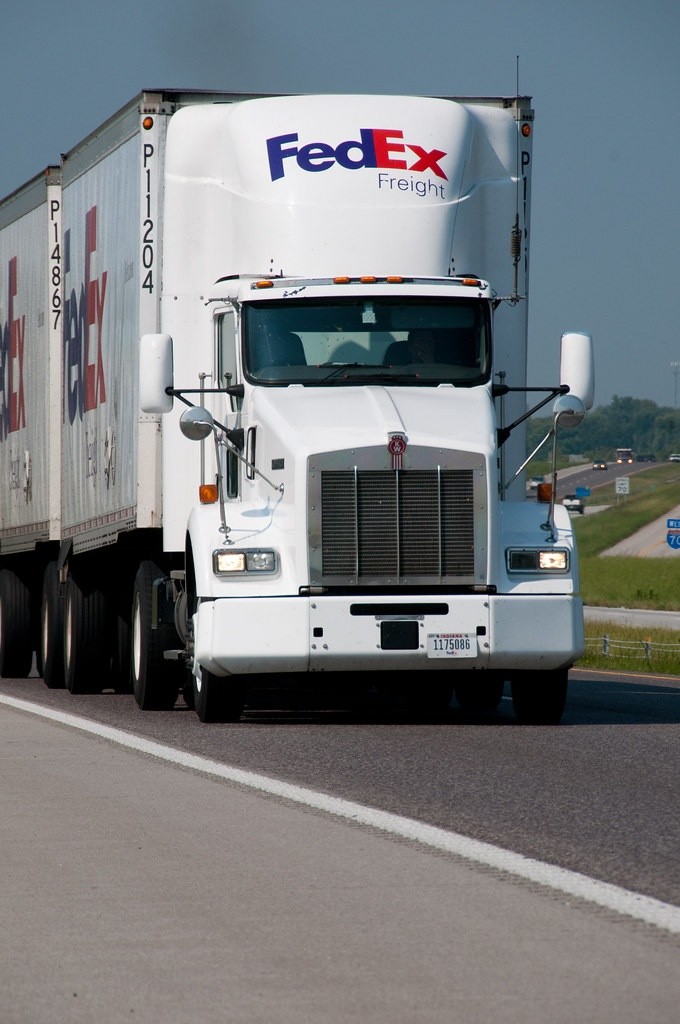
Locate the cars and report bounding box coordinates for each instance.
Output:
[593,460,607,470]
[529,475,546,489]
[562,495,585,515]
[668,454,680,462]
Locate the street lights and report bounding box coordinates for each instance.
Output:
[670,361,680,409]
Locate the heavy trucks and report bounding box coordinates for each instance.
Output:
[1,84,596,724]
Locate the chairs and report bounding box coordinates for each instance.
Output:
[381,340,433,366]
[255,331,307,370]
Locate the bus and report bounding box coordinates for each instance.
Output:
[617,448,636,464]
